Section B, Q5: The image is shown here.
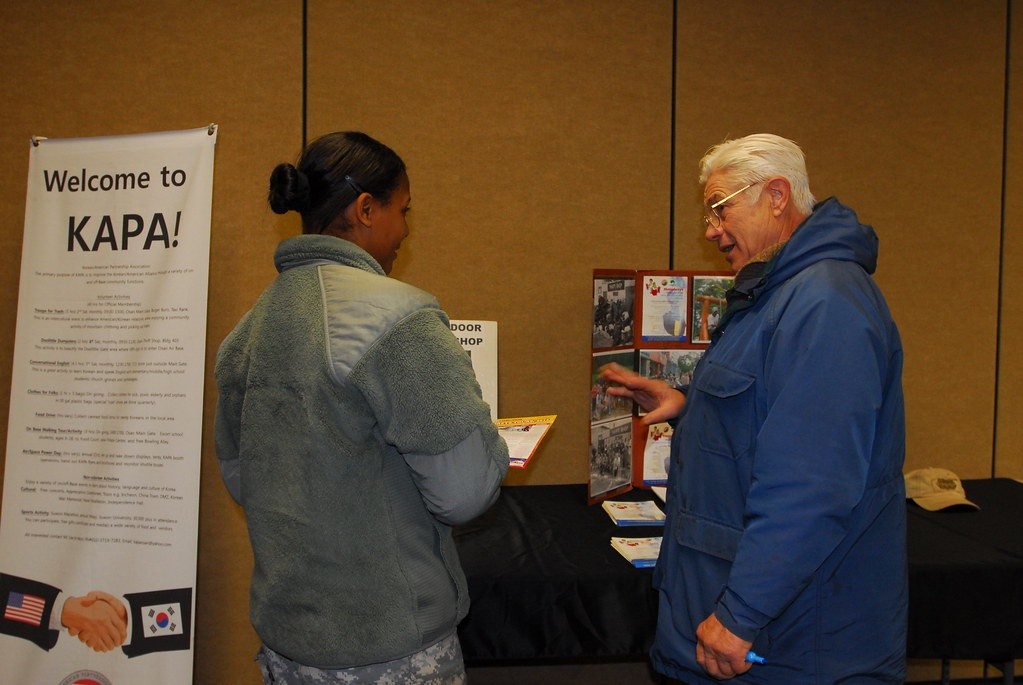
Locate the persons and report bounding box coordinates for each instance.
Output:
[213,130,510,685]
[592,380,634,422]
[708,307,719,335]
[592,441,631,482]
[601,133,909,685]
[595,294,634,346]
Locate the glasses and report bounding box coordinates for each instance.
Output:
[703,181,764,228]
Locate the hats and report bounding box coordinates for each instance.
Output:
[904,468,979,511]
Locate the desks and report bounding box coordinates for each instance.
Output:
[453,475,1023,685]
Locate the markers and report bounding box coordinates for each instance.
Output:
[745,652,770,665]
